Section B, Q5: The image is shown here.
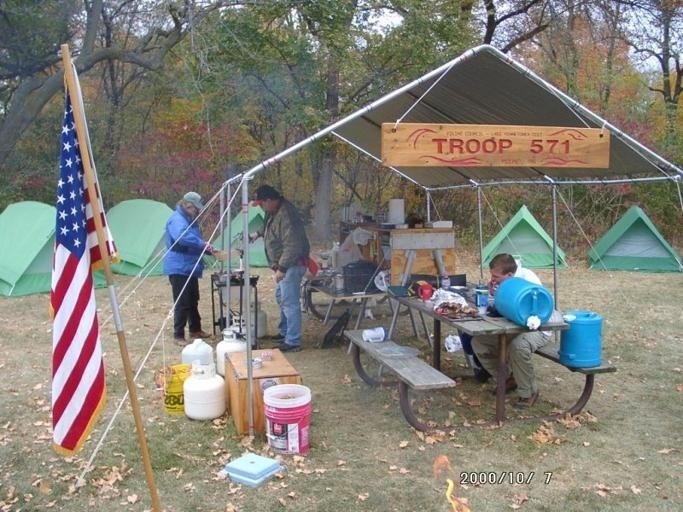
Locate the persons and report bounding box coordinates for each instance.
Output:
[249,185,310,353]
[163,191,227,347]
[470,252,554,409]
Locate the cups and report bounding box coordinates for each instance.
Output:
[417,286,432,300]
[476,289,488,315]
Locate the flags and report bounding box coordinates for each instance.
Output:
[51,65,117,463]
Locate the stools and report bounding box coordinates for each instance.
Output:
[314,286,356,301]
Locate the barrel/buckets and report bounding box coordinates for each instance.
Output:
[493,277,553,329]
[558,309,605,368]
[164,364,193,416]
[263,384,311,454]
[182,338,214,365]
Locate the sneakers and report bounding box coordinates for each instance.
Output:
[189,330,212,338]
[271,333,301,352]
[513,389,539,410]
[174,338,188,346]
[492,372,517,395]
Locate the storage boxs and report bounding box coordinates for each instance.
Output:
[224,348,300,436]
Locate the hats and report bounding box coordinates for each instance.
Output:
[252,184,274,207]
[184,192,204,209]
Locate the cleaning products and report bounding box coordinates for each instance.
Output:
[439,271,451,290]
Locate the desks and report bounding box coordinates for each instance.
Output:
[211,273,258,347]
[356,297,594,433]
[340,223,455,287]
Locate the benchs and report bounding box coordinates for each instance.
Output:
[344,327,458,388]
[537,346,617,375]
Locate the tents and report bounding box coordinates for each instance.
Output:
[105,198,216,276]
[479,203,568,270]
[211,199,270,268]
[586,205,683,273]
[0,201,115,297]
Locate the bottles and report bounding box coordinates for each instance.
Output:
[476,279,485,289]
[377,207,384,228]
[335,273,344,297]
[362,327,388,343]
[321,256,327,270]
